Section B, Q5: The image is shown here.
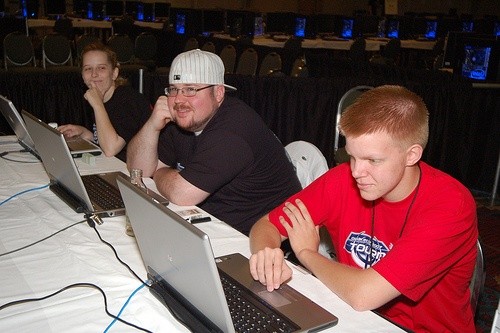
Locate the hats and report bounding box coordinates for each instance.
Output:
[169,49,237,91]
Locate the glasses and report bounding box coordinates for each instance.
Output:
[164,84,216,98]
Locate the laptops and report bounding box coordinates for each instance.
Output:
[0,94,103,158]
[114,175,338,332]
[20,110,169,219]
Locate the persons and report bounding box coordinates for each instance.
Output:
[126,49,304,238]
[54,42,153,166]
[249,86,479,333]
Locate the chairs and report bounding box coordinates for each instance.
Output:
[3,30,449,82]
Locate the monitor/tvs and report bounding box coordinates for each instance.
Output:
[19,0,500,83]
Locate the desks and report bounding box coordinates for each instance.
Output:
[0,68,500,191]
[0,136,409,333]
